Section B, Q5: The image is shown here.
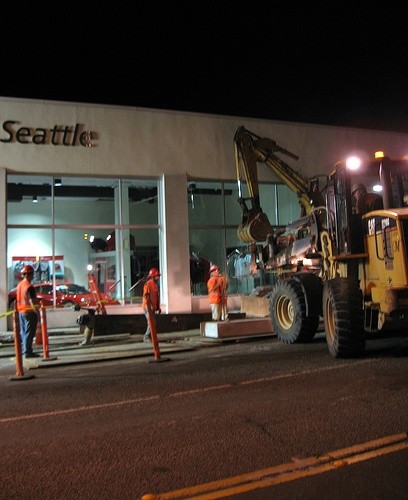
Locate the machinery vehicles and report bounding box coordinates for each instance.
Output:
[230,123,408,361]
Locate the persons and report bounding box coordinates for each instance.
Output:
[352,188,382,212]
[17,265,41,358]
[207,265,228,320]
[142,268,162,343]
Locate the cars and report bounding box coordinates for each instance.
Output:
[8,280,121,312]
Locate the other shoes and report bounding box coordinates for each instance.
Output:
[26,353,39,357]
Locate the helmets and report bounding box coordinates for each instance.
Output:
[148,268,159,277]
[209,265,218,273]
[21,265,34,273]
[352,183,364,194]
